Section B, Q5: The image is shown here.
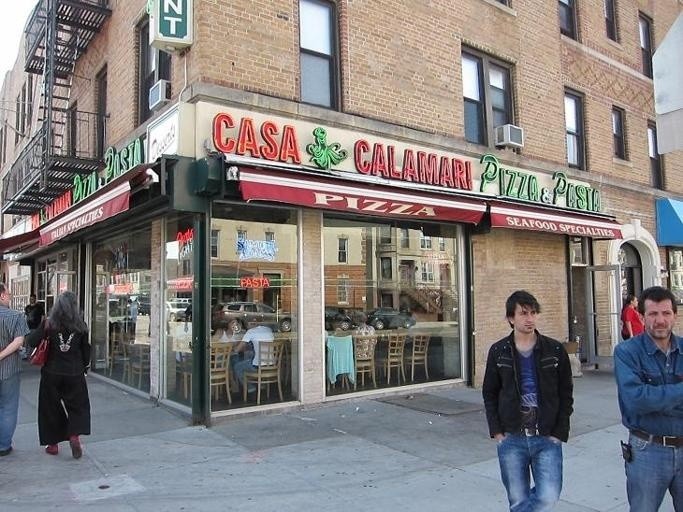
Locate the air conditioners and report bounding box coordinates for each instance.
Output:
[497,124,525,148]
[148,79,170,111]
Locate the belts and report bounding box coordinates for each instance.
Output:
[629,427,683,448]
[518,427,541,438]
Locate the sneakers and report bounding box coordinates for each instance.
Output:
[0,446,13,456]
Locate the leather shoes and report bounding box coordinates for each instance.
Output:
[70,435,83,459]
[45,443,59,454]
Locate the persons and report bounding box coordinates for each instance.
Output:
[171,309,192,374]
[621,295,645,341]
[615,286,682,512]
[483,290,573,512]
[22,294,45,360]
[128,299,138,323]
[183,304,192,332]
[236,314,275,401]
[0,282,26,455]
[26,291,91,459]
[333,312,378,387]
[210,315,246,392]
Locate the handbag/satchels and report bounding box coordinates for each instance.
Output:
[28,319,50,367]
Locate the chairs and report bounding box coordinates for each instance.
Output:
[210,343,232,404]
[242,340,291,405]
[172,335,192,403]
[109,325,150,390]
[326,329,439,394]
